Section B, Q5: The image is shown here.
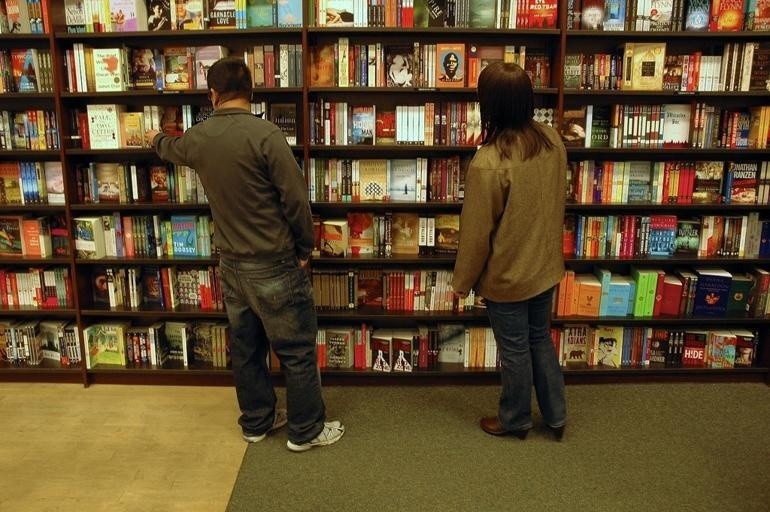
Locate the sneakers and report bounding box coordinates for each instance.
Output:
[288,420,344,452]
[242,408,288,442]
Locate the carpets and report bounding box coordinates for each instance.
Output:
[224,382,770,512]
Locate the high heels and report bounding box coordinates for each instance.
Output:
[481,417,529,440]
[546,425,565,440]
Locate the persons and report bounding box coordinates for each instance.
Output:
[144,56,347,451]
[451,62,572,444]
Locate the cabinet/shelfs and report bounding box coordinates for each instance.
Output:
[0,1,769,388]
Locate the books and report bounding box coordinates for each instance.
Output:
[314,322,502,370]
[307,1,561,90]
[1,97,301,204]
[1,210,225,310]
[561,102,768,205]
[308,98,485,203]
[551,211,768,318]
[550,322,761,371]
[0,319,230,369]
[1,0,302,93]
[310,210,486,314]
[563,0,769,92]
